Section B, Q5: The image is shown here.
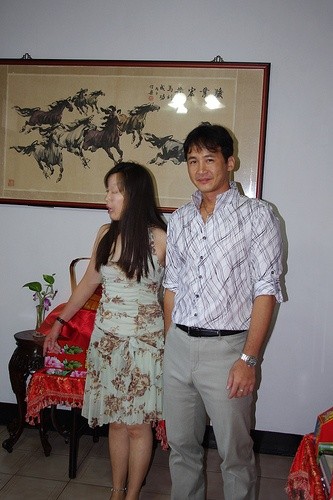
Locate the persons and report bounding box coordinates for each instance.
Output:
[44,163,167,500]
[162,123,284,500]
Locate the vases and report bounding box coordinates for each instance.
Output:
[32,305,46,337]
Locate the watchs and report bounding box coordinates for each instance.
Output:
[240,352,257,366]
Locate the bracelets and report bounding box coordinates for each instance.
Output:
[57,317,66,325]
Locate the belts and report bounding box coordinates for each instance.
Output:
[175,323,248,337]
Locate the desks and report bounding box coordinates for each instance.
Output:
[2,330,69,457]
[25,369,169,479]
[283,433,326,500]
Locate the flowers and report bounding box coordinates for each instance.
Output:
[21,273,57,312]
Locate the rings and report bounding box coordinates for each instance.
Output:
[240,390,243,391]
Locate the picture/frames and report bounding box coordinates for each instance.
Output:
[0,59,271,214]
